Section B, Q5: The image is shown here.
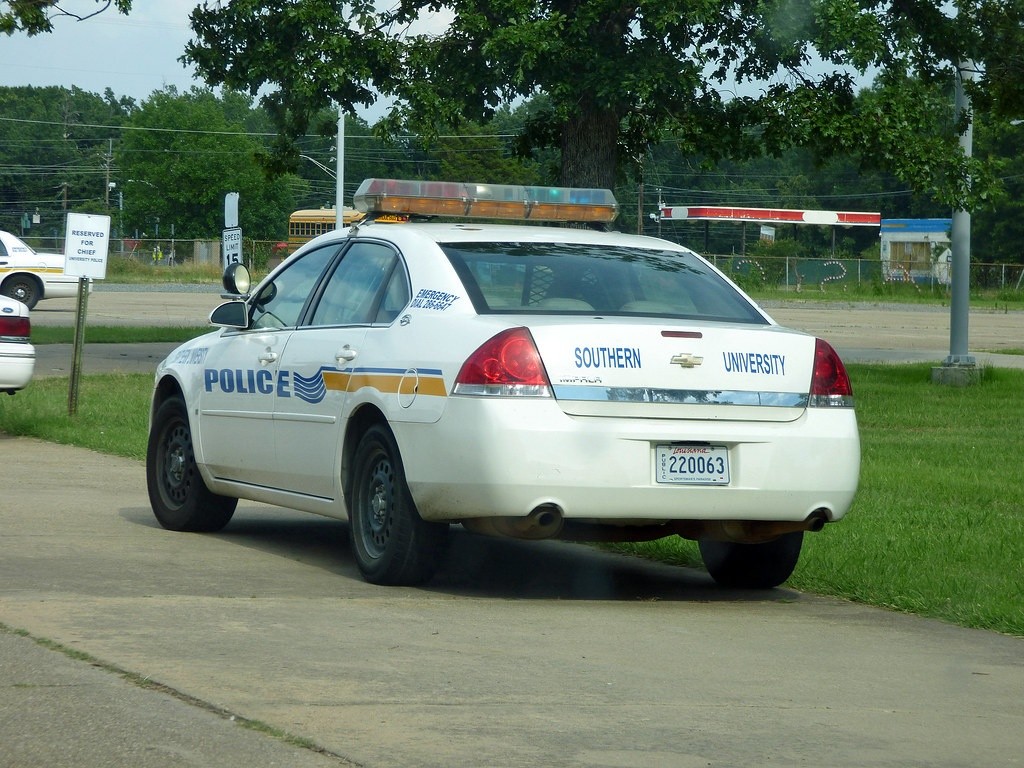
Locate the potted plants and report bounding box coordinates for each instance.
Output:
[266,248,291,273]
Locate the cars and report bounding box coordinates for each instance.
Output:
[145,179,861,601]
[0,232,94,311]
[0,295,36,395]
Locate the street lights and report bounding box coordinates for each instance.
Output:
[285,149,345,232]
[104,182,116,208]
[128,180,159,265]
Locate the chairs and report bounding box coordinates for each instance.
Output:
[537,298,595,311]
[618,300,675,313]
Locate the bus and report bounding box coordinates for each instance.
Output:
[288,205,410,259]
